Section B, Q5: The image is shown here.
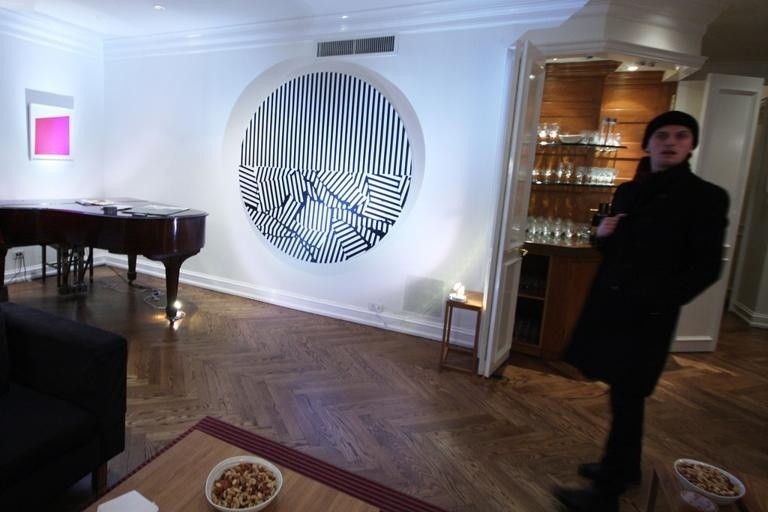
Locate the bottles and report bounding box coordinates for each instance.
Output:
[599,117,617,146]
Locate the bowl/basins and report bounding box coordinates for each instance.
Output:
[203,454,283,512]
[559,134,583,144]
[673,458,747,507]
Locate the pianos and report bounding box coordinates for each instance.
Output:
[1,198,209,326]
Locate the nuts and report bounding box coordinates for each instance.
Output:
[210,462,278,508]
[677,461,740,496]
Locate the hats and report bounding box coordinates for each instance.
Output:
[642,111,699,150]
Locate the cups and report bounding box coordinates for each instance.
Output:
[576,167,618,186]
[525,215,590,244]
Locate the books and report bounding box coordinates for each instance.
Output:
[74,198,115,207]
[123,205,190,217]
[100,204,132,211]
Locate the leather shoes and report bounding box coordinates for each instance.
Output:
[550,462,642,511]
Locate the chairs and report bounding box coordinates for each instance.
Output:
[0,299,126,512]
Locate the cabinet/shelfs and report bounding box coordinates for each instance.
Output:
[531,143,625,189]
[509,249,552,356]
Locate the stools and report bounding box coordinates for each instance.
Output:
[440,288,484,376]
[42,243,94,285]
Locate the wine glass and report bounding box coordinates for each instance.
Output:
[530,162,573,187]
[537,122,560,146]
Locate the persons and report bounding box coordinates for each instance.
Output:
[550,111,731,511]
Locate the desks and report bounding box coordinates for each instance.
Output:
[651,449,768,512]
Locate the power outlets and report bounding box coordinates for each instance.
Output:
[12,251,24,259]
